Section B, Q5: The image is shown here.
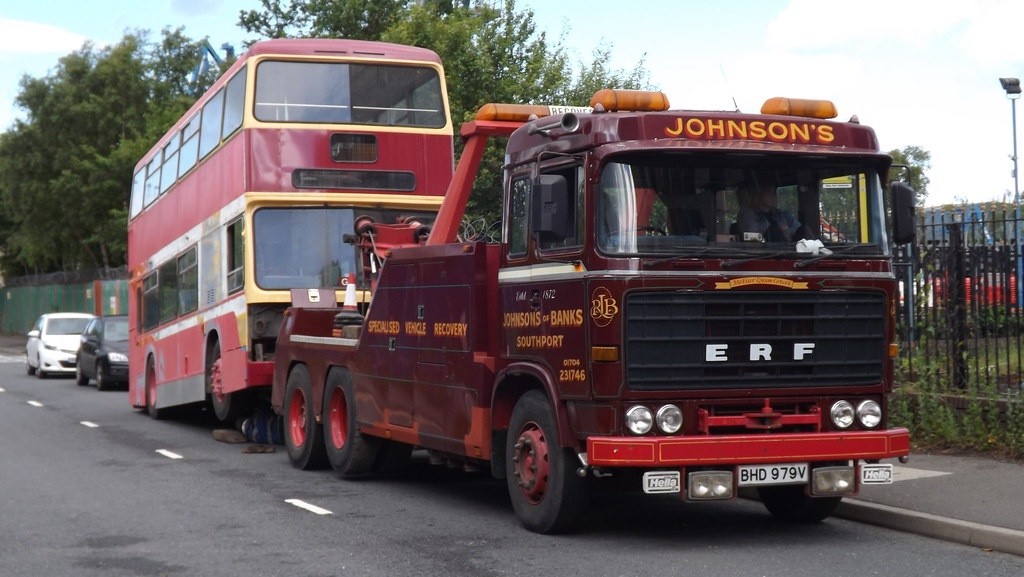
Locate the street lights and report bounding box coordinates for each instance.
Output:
[999,75,1024,324]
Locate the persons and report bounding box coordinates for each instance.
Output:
[737,174,801,242]
[235,408,284,445]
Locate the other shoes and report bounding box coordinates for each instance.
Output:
[242,446,275,453]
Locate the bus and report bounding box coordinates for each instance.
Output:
[128,38,456,425]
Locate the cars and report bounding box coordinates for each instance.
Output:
[75,314,129,391]
[25,312,99,380]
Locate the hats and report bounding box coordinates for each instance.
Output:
[739,163,779,190]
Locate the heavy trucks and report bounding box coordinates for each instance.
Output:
[269,90,917,535]
